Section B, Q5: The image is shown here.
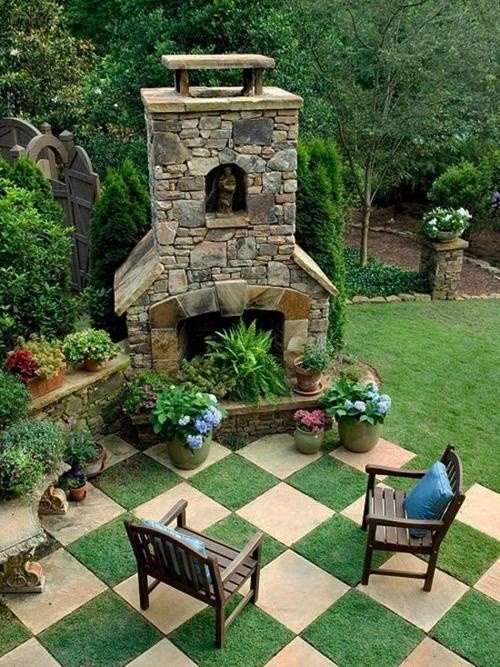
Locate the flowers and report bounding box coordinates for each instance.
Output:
[66,463,85,488]
[62,328,120,366]
[4,333,67,381]
[294,409,325,433]
[318,376,392,426]
[423,206,472,239]
[66,418,97,460]
[302,334,336,371]
[150,382,230,455]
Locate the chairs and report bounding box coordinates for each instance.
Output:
[362,443,466,591]
[124,499,264,649]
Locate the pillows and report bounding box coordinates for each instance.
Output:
[144,519,212,587]
[403,461,454,538]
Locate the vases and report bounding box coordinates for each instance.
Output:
[16,371,64,399]
[294,355,322,391]
[338,417,380,453]
[294,423,324,455]
[80,442,104,474]
[437,229,465,243]
[168,432,212,469]
[70,480,86,499]
[85,358,106,372]
